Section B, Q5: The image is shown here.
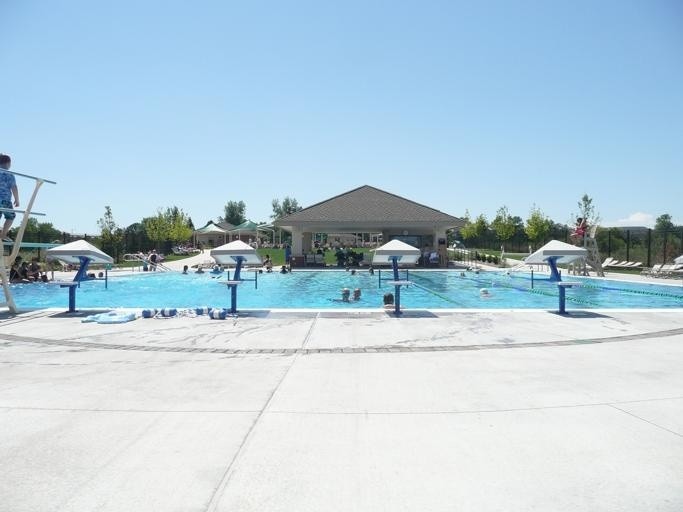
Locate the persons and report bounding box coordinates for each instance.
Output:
[0,153,20,242]
[210,265,221,274]
[349,288,361,301]
[345,266,349,272]
[279,265,288,273]
[178,245,183,252]
[8,255,43,283]
[381,292,404,309]
[368,267,374,275]
[316,246,322,254]
[350,269,357,275]
[285,243,292,264]
[135,249,165,272]
[479,288,491,298]
[332,288,350,302]
[181,265,188,274]
[499,243,505,259]
[194,264,205,273]
[569,217,587,246]
[262,255,273,272]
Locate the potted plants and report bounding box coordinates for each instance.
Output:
[477,254,499,264]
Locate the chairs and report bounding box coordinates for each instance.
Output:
[576,256,683,279]
[315,254,326,267]
[306,255,315,267]
[171,247,201,256]
